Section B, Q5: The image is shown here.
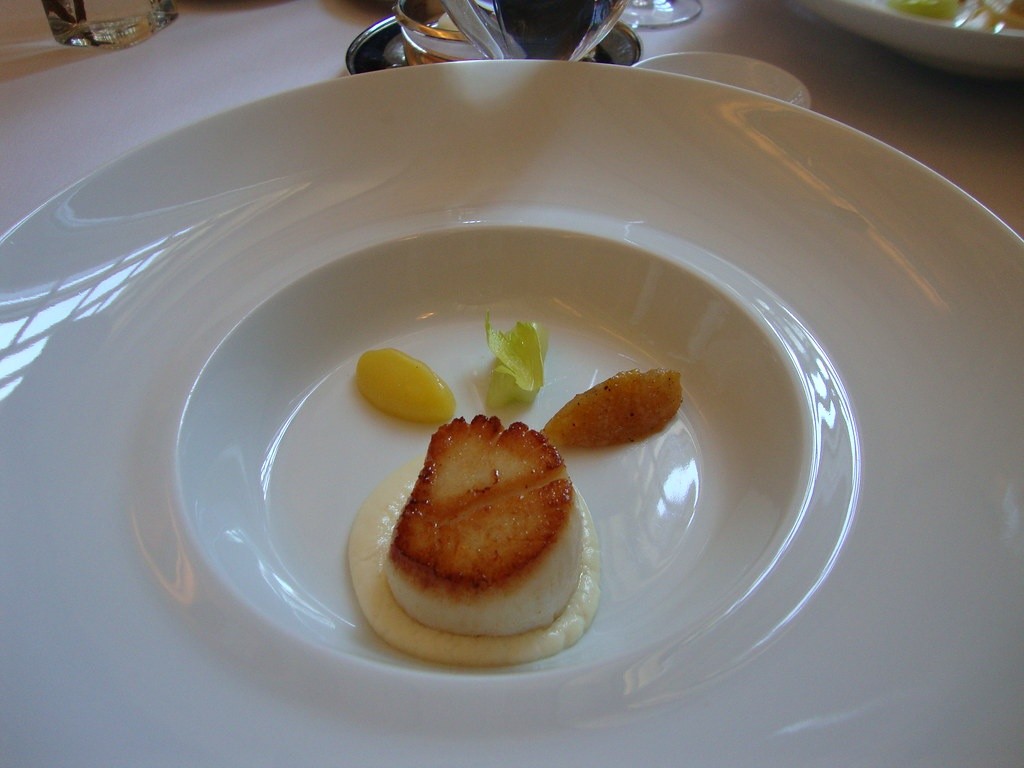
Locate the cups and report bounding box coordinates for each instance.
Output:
[40,0,176,51]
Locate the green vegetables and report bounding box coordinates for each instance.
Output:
[485,307,546,392]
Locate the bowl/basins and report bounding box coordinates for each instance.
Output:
[785,0,1024,59]
[394,0,484,65]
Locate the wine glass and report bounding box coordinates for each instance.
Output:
[620,0,701,28]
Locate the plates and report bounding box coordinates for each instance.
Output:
[345,14,641,75]
[630,53,811,112]
[0,60,1024,768]
